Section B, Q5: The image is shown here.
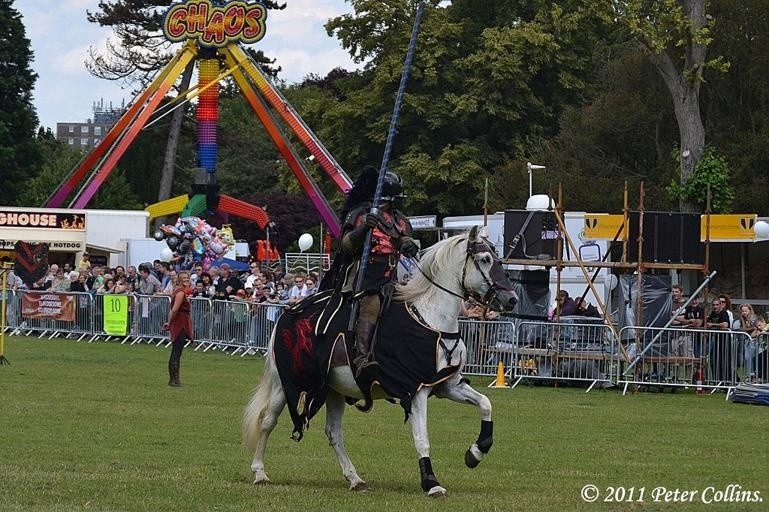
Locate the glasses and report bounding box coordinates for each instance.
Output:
[306,283,313,286]
[250,266,257,268]
[295,280,303,283]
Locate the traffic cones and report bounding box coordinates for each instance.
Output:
[492,362,512,391]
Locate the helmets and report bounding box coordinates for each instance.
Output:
[375,171,403,195]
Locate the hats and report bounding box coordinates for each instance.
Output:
[236,289,246,296]
[220,263,234,274]
[82,252,90,257]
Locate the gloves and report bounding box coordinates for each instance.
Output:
[400,239,419,259]
[359,212,382,233]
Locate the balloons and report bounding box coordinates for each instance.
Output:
[298,233,314,252]
[754,220,768,240]
[154,217,236,272]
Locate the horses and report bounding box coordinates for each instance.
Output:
[241,223,520,500]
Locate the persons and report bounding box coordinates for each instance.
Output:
[339,166,419,380]
[0,250,767,394]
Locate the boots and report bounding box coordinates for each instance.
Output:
[353,321,381,379]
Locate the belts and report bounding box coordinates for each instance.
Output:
[372,254,399,268]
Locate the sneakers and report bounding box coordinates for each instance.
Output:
[168,381,184,387]
[697,381,702,390]
